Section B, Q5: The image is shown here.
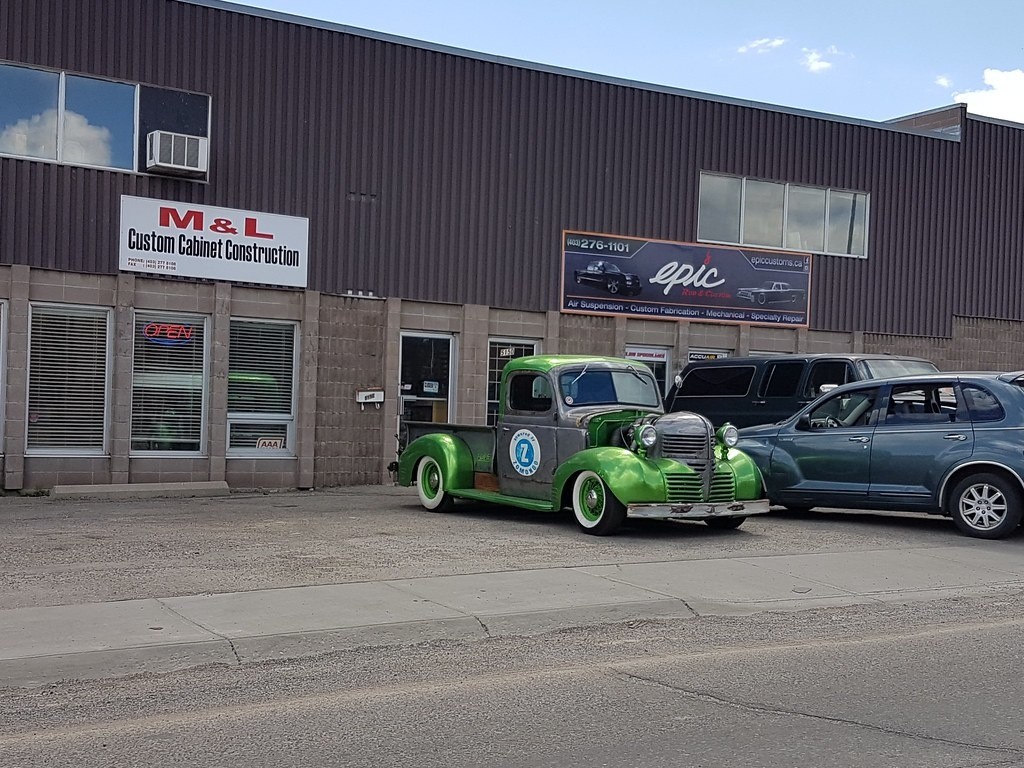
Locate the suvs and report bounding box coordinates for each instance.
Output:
[663,355,976,435]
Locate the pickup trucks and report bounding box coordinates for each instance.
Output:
[394,352,771,536]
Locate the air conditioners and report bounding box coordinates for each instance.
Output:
[145,130,208,176]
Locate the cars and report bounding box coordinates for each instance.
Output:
[575,260,643,297]
[731,371,1024,541]
[736,280,806,305]
